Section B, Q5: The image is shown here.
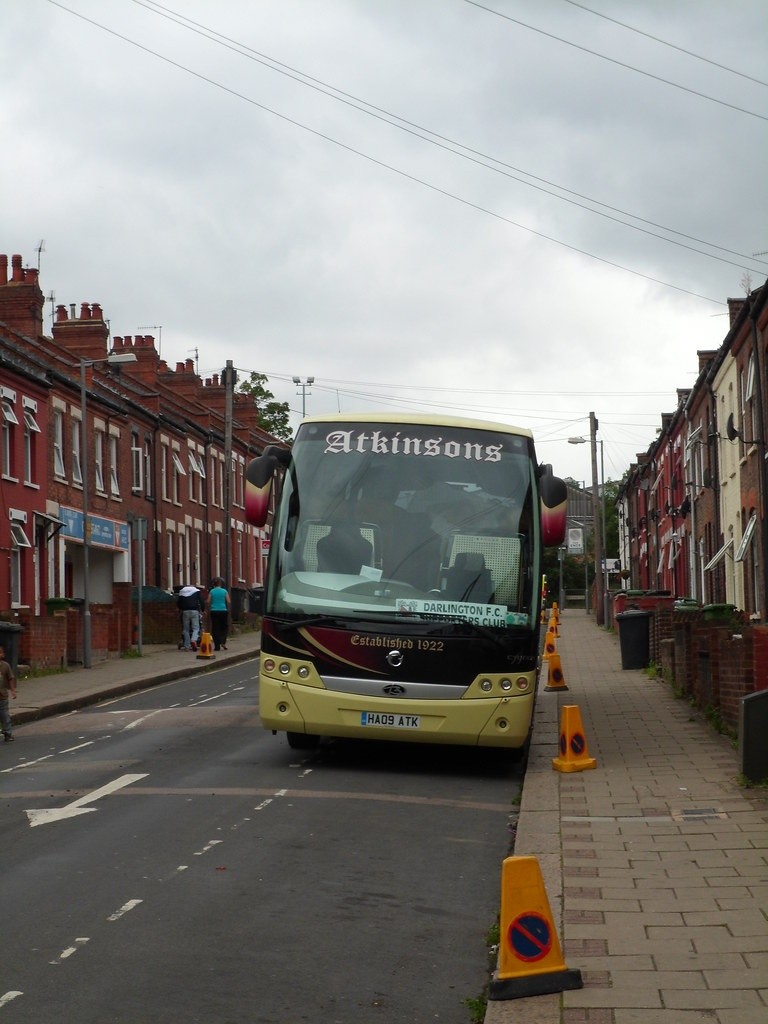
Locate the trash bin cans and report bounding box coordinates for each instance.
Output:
[618,588,671,596]
[673,598,700,622]
[702,602,738,619]
[232,586,247,624]
[0,621,26,689]
[616,610,652,670]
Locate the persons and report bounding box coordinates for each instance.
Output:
[206,578,231,650]
[175,582,206,652]
[317,511,372,576]
[0,646,17,741]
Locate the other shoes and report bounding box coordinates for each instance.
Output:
[191,640,197,651]
[221,643,228,650]
[4,734,13,741]
[214,648,220,651]
[185,648,189,652]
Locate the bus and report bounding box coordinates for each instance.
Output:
[245,409,572,763]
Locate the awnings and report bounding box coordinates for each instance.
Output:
[33,510,67,541]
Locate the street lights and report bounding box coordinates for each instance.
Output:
[563,477,590,613]
[568,436,610,632]
[79,353,140,667]
[293,376,316,417]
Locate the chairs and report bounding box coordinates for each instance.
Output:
[447,551,495,603]
[317,523,374,576]
